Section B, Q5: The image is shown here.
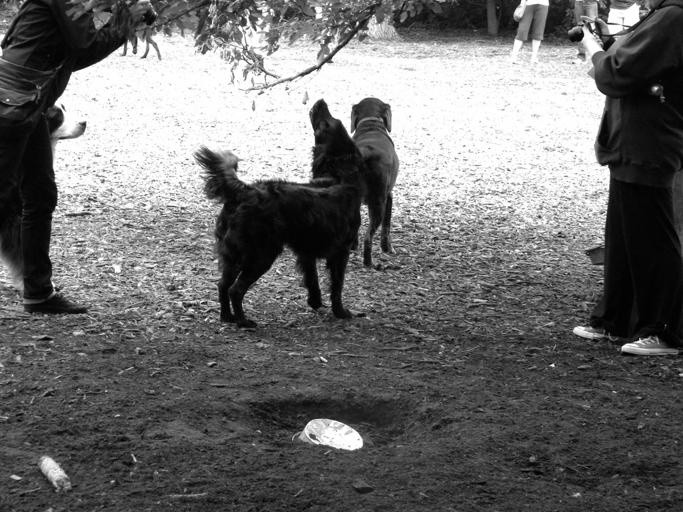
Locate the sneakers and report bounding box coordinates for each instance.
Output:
[23,289,89,315]
[571,57,588,65]
[572,325,635,344]
[620,335,679,356]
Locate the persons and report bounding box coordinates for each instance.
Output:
[566,0,682,356]
[508,0,550,64]
[1,1,155,312]
[574,0,598,62]
[603,0,640,44]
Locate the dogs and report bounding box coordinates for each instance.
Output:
[0,100,87,297]
[350,97,401,267]
[190,98,366,330]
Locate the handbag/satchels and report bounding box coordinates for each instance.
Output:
[0,56,61,142]
[513,0,527,22]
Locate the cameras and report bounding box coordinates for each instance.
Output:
[568,21,602,42]
[128,0,157,25]
